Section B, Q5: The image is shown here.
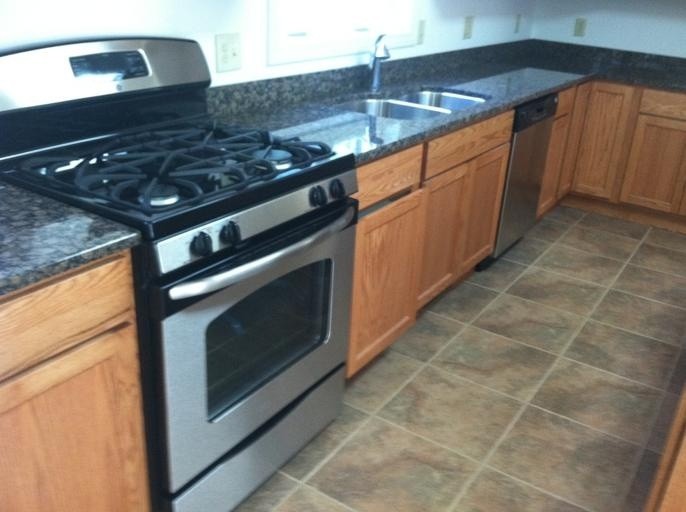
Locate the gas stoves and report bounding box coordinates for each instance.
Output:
[0,114,357,239]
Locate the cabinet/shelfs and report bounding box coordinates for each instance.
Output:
[0,249,152,512]
[535,78,684,222]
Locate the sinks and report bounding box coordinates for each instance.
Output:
[398,90,485,113]
[334,98,450,121]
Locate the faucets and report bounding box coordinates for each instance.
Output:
[372,34,391,92]
[364,113,385,146]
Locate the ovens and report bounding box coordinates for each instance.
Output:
[140,153,358,511]
